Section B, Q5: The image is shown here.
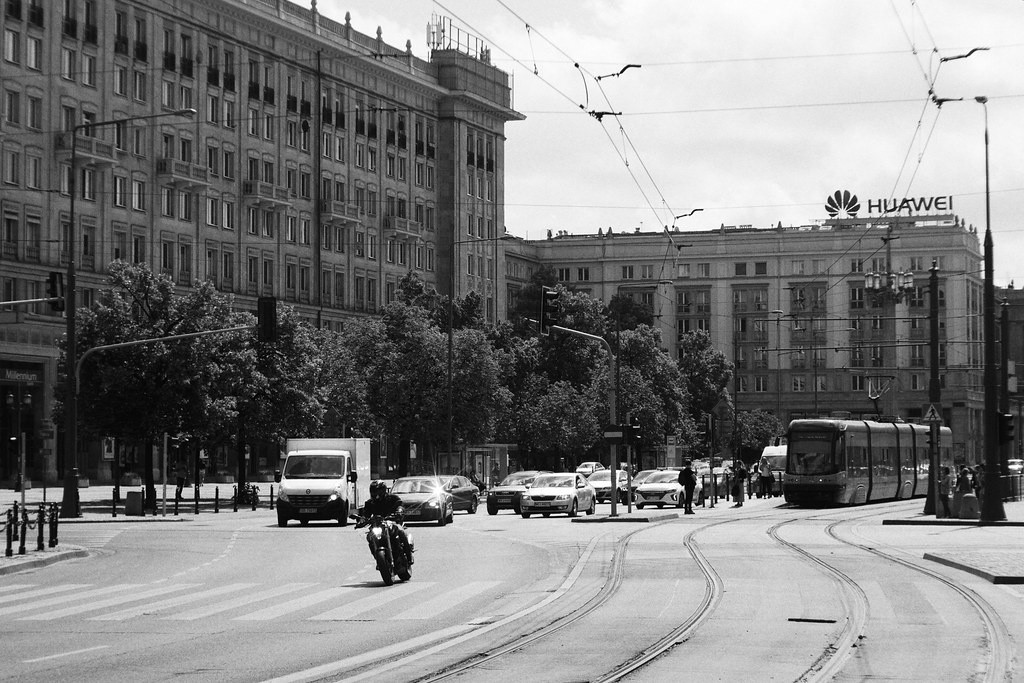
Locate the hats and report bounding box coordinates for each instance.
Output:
[736,460,742,465]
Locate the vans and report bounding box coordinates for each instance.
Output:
[754,444,789,494]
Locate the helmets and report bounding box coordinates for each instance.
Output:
[370,480,387,501]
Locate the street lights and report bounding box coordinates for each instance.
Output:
[447,236,515,472]
[59,107,198,519]
[614,279,674,469]
[975,95,1008,520]
[734,309,784,461]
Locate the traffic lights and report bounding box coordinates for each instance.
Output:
[999,413,1014,444]
[539,285,560,337]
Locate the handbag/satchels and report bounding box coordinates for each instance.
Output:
[768,470,776,484]
[731,479,740,497]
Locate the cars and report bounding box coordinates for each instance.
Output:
[371,474,481,515]
[608,456,759,510]
[576,461,605,478]
[487,470,556,515]
[389,476,454,527]
[520,472,597,519]
[586,469,634,504]
[1007,458,1024,475]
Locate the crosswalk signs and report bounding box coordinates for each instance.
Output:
[922,404,943,421]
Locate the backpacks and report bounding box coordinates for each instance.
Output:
[678,470,693,486]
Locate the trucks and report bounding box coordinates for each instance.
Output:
[274,438,372,527]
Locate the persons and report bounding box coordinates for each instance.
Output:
[471,470,486,496]
[731,461,746,506]
[937,466,953,519]
[356,480,414,570]
[678,461,697,514]
[758,457,776,499]
[200,460,207,484]
[174,458,188,499]
[954,468,971,492]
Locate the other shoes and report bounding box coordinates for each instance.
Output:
[685,510,695,514]
[406,552,414,566]
[376,563,379,570]
[735,504,743,507]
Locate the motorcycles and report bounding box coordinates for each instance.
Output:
[348,499,416,586]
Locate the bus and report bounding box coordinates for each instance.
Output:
[774,415,954,507]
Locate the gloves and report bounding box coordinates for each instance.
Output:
[356,517,367,529]
[394,511,403,523]
